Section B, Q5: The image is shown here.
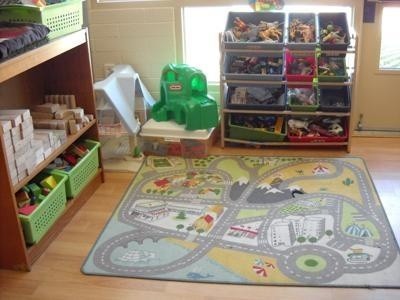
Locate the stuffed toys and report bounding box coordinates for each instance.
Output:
[223,14,349,137]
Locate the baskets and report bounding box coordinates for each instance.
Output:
[0,0,83,41]
[17,171,68,245]
[50,137,101,198]
[220,11,350,143]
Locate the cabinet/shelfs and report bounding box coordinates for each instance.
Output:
[217,10,359,154]
[0,25,106,273]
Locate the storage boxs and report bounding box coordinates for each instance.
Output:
[139,118,216,160]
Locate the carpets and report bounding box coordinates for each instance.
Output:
[80,153,400,290]
